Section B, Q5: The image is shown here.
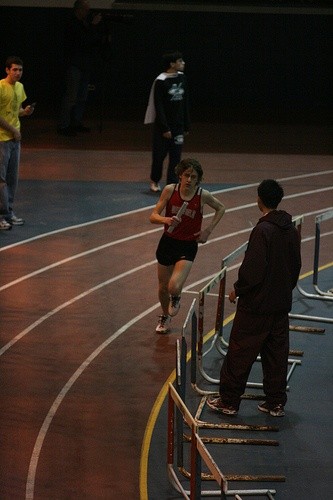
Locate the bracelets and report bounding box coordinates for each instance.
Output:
[205,230,211,234]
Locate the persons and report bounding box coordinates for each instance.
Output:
[145,52,190,193]
[58,0,103,137]
[150,158,225,334]
[206,179,301,417]
[0,56,35,230]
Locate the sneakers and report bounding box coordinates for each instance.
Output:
[205,395,238,415]
[257,400,286,416]
[0,219,12,230]
[155,314,173,334]
[11,215,25,224]
[168,294,181,316]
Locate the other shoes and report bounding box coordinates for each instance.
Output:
[150,183,162,192]
